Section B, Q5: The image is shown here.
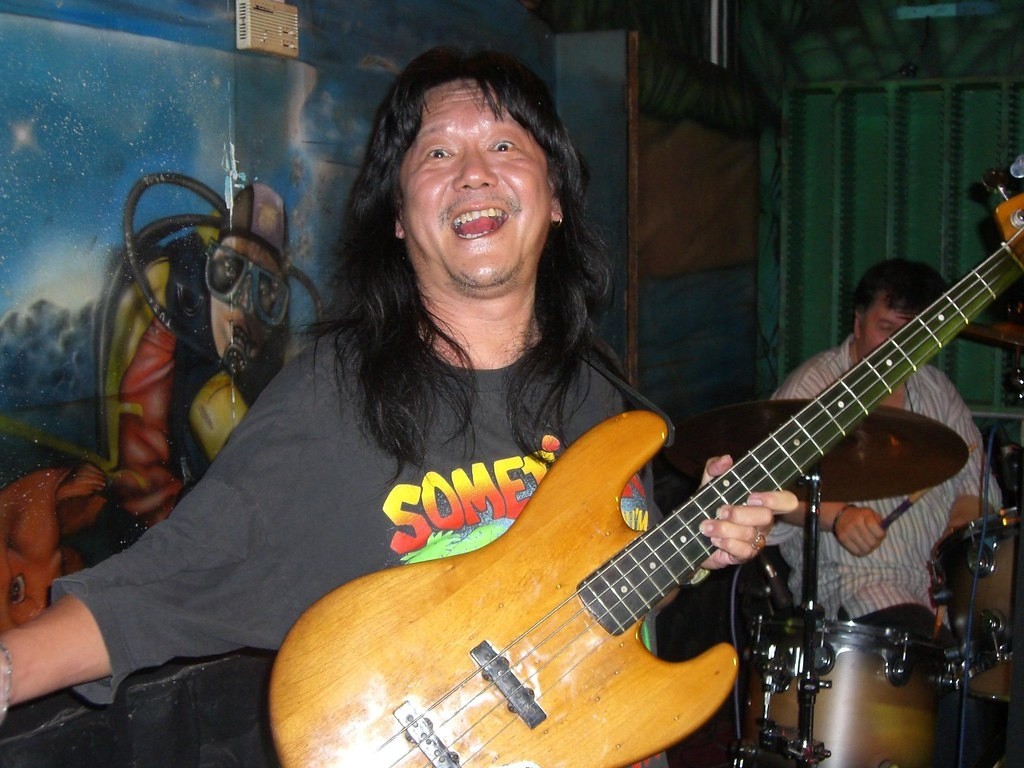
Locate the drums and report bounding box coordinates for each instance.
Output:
[929,504,1019,705]
[732,607,951,768]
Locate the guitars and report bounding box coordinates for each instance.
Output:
[268,154,1024,768]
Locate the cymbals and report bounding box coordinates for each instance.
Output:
[665,399,969,503]
[954,321,1023,351]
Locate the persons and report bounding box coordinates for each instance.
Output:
[0,43,799,768]
[766,259,1003,645]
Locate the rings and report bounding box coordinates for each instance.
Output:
[752,526,767,549]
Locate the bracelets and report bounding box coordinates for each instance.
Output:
[0,644,12,724]
[832,504,857,536]
